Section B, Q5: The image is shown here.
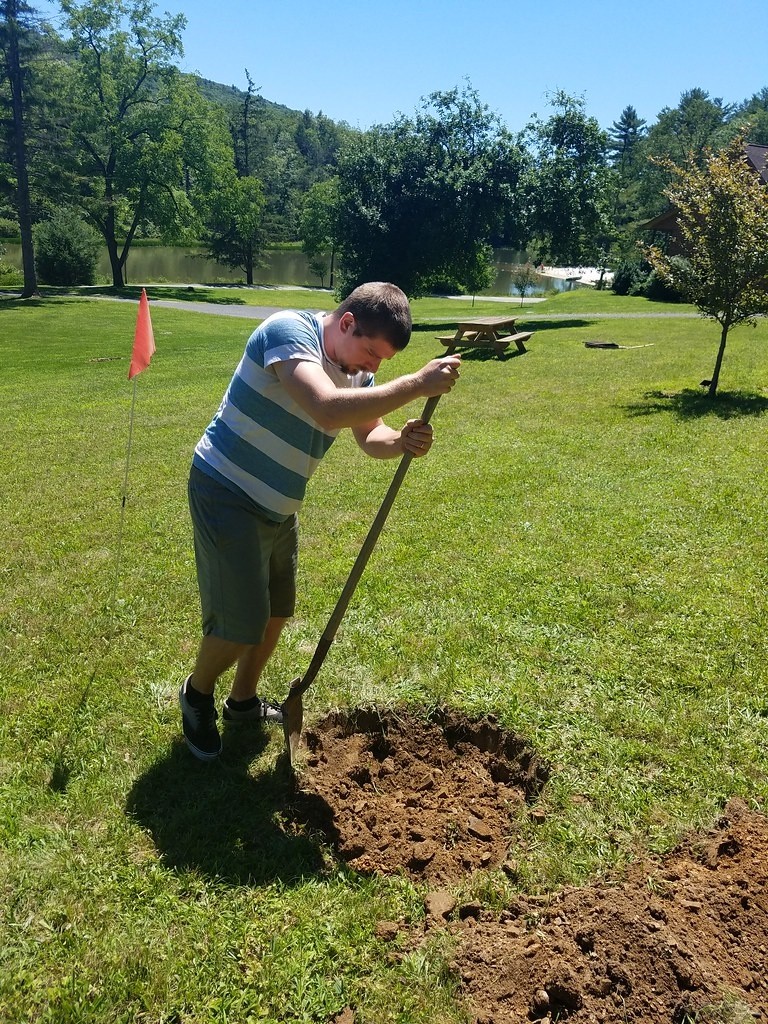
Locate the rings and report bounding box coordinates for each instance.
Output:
[420,442,424,449]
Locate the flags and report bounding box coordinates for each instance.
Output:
[128,288,156,380]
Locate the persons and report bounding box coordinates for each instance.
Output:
[178,281,461,763]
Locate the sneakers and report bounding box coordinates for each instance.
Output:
[179,672,222,762]
[222,697,284,726]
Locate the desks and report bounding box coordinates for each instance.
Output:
[444,316,527,361]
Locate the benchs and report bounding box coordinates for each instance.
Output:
[435,331,478,346]
[495,332,535,348]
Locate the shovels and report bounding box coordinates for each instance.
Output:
[279,395,442,768]
